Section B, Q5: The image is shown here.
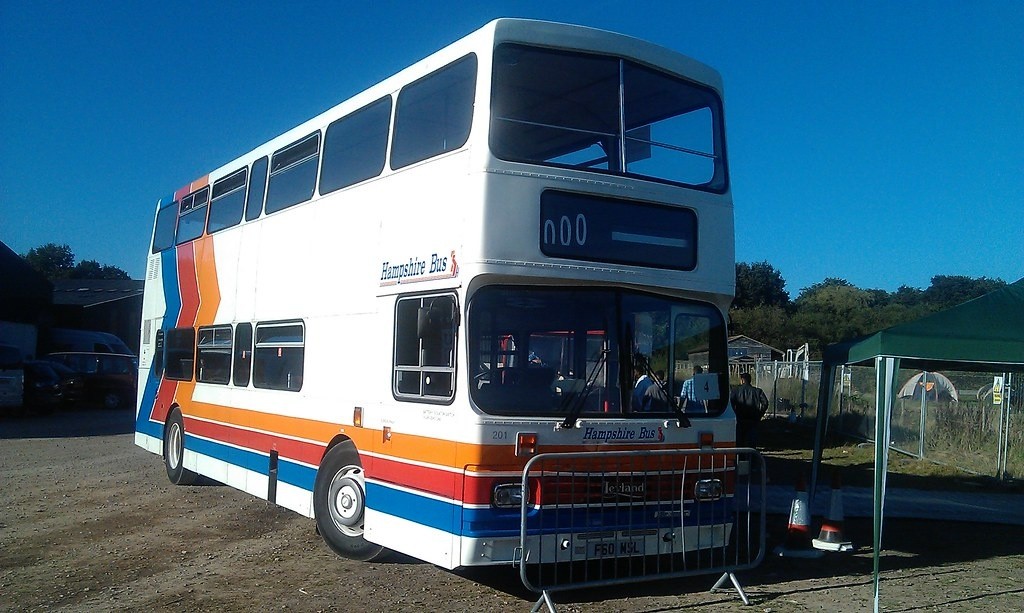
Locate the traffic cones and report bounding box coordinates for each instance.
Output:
[787,406,797,430]
[812,474,853,552]
[786,477,811,543]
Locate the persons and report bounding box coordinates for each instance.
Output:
[679,365,706,413]
[731,373,769,448]
[633,367,654,411]
[641,370,668,412]
[529,350,541,363]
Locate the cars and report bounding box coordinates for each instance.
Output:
[0,323,139,413]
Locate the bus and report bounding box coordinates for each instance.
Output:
[133,22,739,570]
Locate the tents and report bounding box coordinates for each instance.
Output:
[897,370,958,402]
[807,275,1024,613]
[977,382,1016,405]
